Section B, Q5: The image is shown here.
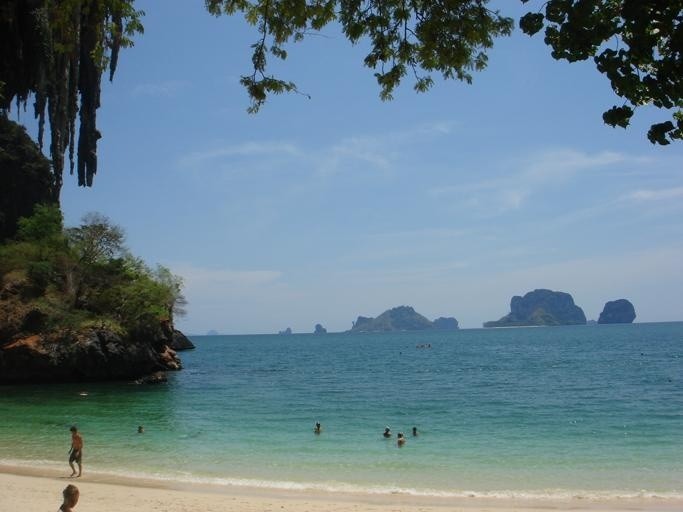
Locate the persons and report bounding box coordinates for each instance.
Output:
[55,484,79,512]
[312,421,322,433]
[67,425,82,477]
[137,426,144,433]
[411,427,419,436]
[382,426,391,438]
[397,433,404,447]
